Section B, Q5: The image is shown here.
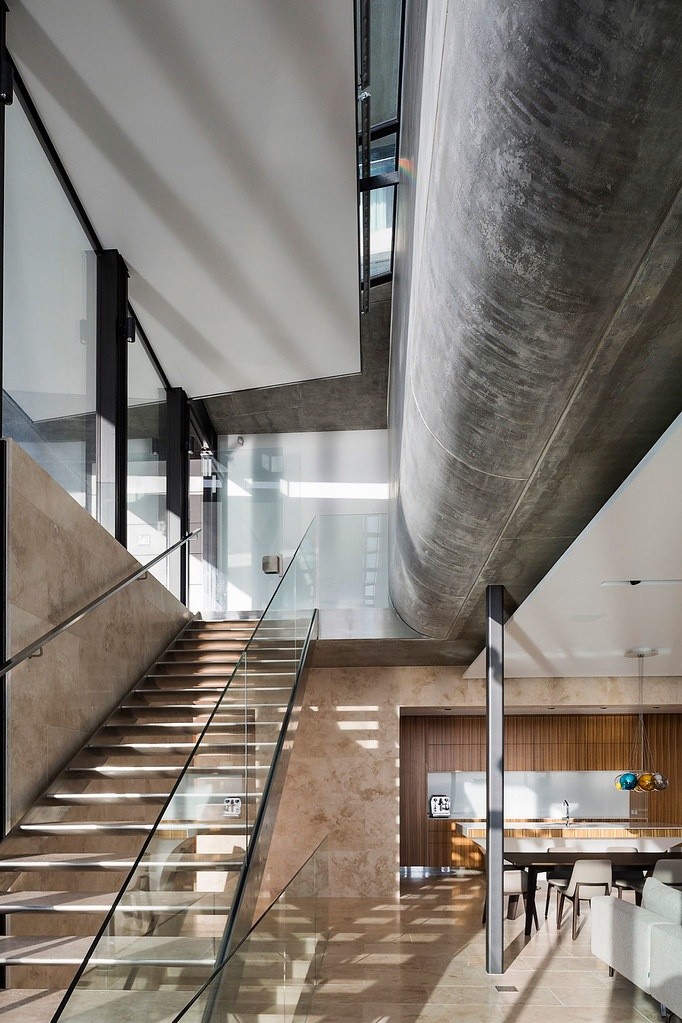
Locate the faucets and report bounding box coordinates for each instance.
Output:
[563,799,570,827]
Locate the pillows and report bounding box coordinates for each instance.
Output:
[640,876,681,926]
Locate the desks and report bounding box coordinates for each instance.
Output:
[482,853,682,936]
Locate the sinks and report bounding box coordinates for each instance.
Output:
[542,822,580,826]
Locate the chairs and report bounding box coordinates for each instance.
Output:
[504,843,682,940]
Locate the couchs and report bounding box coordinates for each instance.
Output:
[590,895,682,1019]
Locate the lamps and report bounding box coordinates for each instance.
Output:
[614,647,669,793]
